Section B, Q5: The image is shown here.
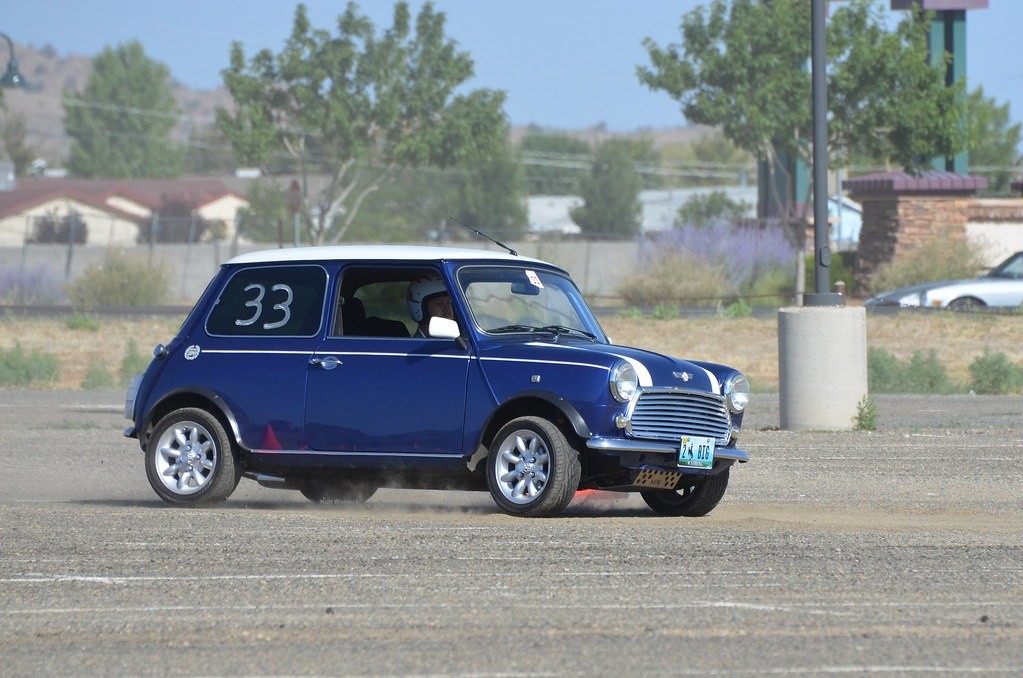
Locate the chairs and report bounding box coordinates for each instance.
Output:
[342,297,410,338]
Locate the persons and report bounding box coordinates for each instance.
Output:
[407,273,454,338]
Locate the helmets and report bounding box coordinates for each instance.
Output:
[407,275,448,323]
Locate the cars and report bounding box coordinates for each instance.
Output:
[864,248,1023,310]
[123,244,753,517]
